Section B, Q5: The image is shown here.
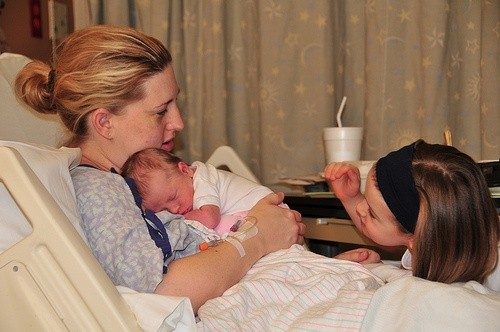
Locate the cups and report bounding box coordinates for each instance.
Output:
[321,126,364,192]
[352,160,378,195]
[475,160,500,188]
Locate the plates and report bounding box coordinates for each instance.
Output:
[302,191,336,199]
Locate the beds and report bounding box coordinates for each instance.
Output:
[0,49,309,332]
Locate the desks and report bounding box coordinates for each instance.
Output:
[300,215,376,247]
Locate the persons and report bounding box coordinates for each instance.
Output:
[16,24,500,332]
[323,138,500,293]
[123,147,292,238]
